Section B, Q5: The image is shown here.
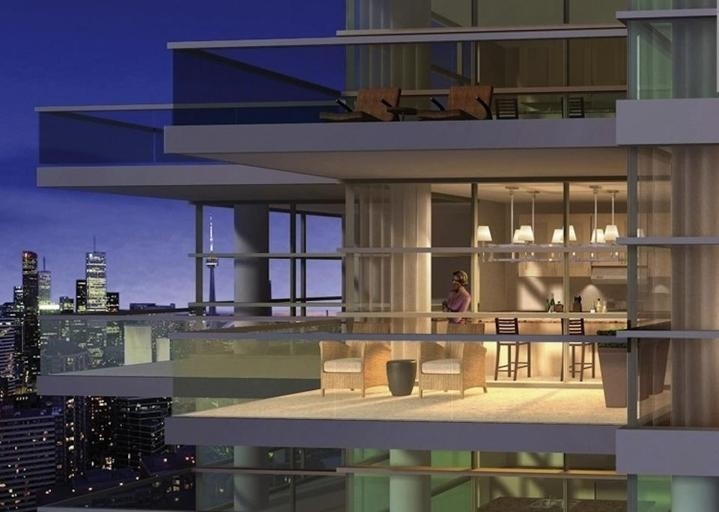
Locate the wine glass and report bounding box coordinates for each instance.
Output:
[441,296,449,307]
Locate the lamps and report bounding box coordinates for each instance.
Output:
[476,185,645,264]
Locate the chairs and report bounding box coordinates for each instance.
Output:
[560,96,585,118]
[320,87,401,122]
[319,321,393,397]
[560,318,595,382]
[417,85,494,120]
[494,98,519,119]
[494,318,531,381]
[418,323,488,399]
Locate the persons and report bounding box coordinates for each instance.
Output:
[442,270,470,323]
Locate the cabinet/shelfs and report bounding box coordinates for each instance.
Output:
[519,212,672,278]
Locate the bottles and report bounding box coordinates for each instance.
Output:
[589,304,596,313]
[551,293,555,305]
[595,298,602,312]
[558,295,561,304]
[545,295,551,311]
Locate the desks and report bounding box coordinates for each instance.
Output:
[385,359,417,396]
[387,107,418,121]
